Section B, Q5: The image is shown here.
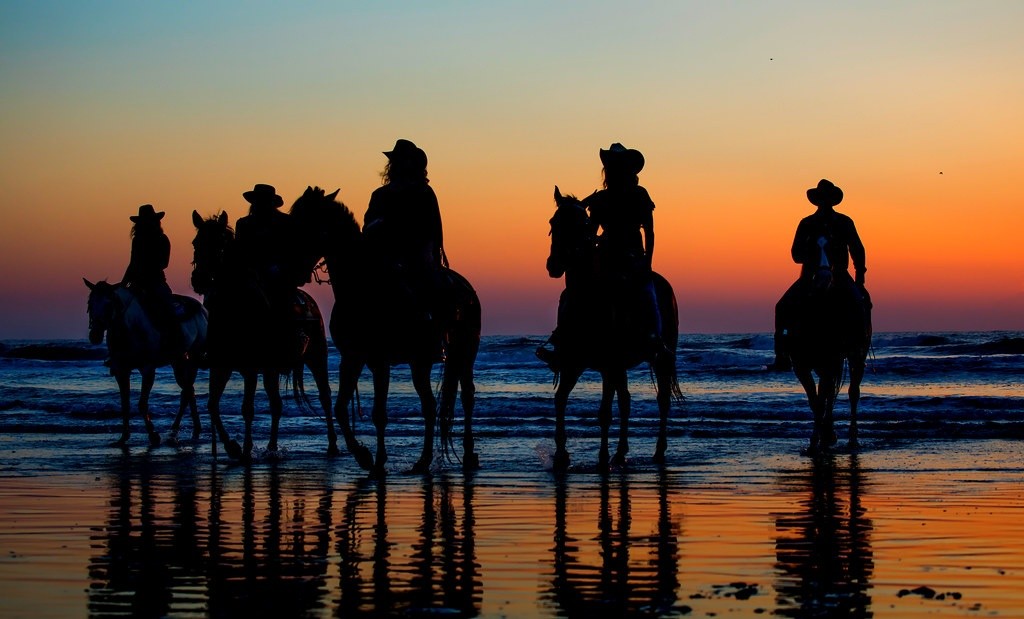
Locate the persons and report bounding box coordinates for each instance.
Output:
[235,184,309,355]
[102,204,189,366]
[773,179,867,372]
[536,143,670,363]
[362,139,449,363]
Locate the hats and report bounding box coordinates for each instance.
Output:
[130,205,165,222]
[383,140,428,167]
[243,184,283,208]
[807,180,843,206]
[600,144,645,173]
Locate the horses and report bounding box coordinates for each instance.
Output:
[786,267,873,457]
[190,208,339,457]
[81,277,208,446]
[544,183,686,469]
[277,186,483,477]
